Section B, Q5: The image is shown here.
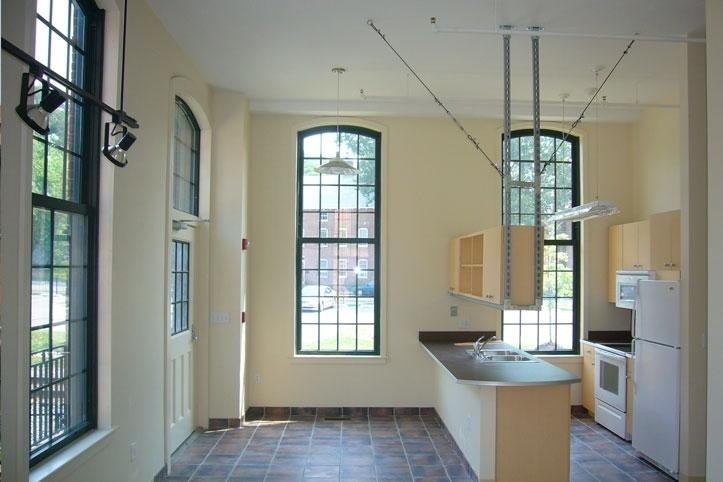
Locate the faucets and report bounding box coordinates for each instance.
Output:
[473,334,496,354]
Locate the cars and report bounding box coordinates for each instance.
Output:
[350,281,374,297]
[300,285,335,312]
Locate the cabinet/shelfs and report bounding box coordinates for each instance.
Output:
[624,353,633,436]
[580,344,595,417]
[446,226,543,309]
[606,220,622,305]
[623,221,649,272]
[650,208,683,281]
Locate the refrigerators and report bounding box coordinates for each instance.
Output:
[632,279,681,479]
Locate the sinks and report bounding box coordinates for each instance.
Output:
[480,350,519,356]
[487,355,531,361]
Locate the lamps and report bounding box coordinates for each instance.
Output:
[544,63,620,224]
[313,68,364,178]
[14,57,136,168]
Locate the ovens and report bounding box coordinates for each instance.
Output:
[593,347,627,440]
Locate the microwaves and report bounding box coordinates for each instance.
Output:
[615,271,656,310]
